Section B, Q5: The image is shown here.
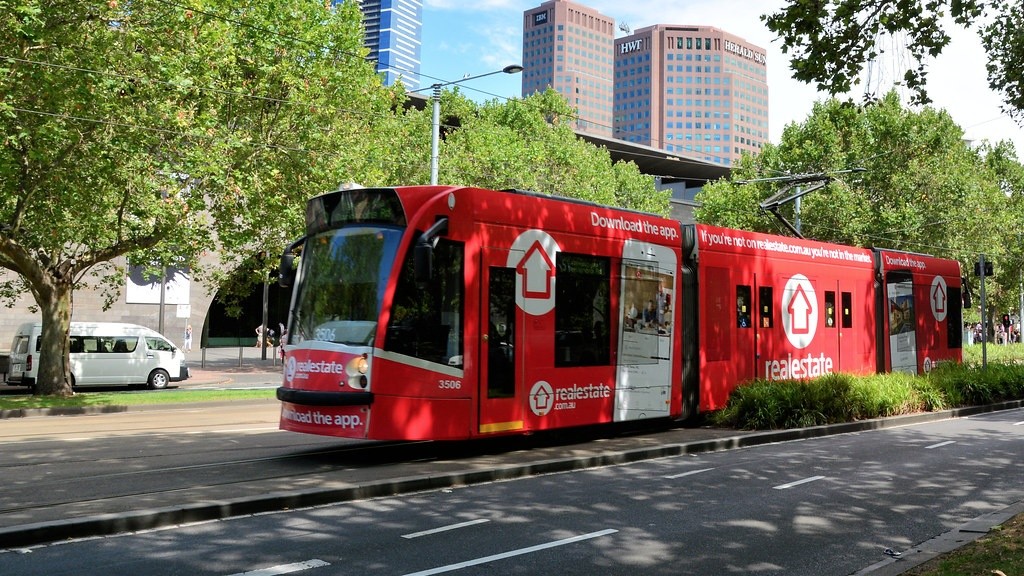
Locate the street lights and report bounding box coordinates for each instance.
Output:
[405,63,523,184]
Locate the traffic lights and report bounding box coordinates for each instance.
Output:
[1003,314,1010,328]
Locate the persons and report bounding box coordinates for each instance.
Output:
[184,325,193,352]
[279,330,288,363]
[255,322,268,347]
[965,321,1021,344]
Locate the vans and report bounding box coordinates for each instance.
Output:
[8,322,189,390]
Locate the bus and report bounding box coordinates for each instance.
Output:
[277,185,971,441]
